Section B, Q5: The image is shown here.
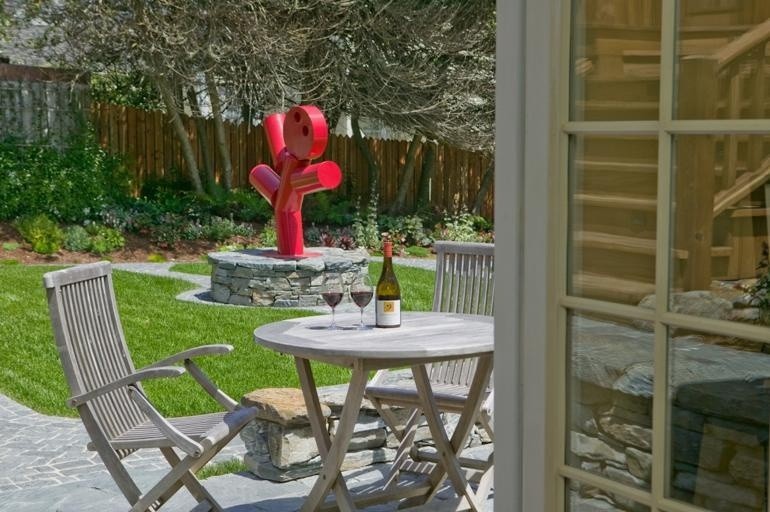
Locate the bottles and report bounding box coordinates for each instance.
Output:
[374,241,401,329]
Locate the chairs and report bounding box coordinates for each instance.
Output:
[42,238,497,512]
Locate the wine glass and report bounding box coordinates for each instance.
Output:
[320,276,343,330]
[350,273,373,328]
